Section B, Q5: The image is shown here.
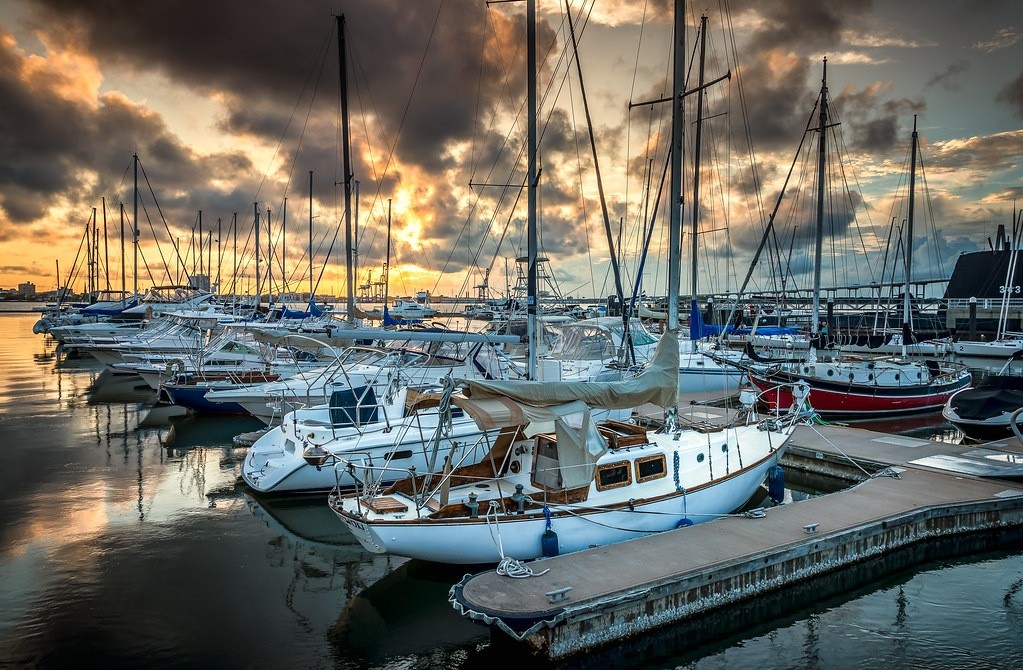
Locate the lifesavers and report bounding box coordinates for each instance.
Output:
[151,310,160,319]
[165,358,184,377]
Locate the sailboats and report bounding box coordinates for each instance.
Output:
[157,171,346,331]
[323,1,811,570]
[239,0,635,495]
[625,15,1023,437]
[35,152,216,332]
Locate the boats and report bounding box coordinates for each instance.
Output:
[48,301,479,423]
